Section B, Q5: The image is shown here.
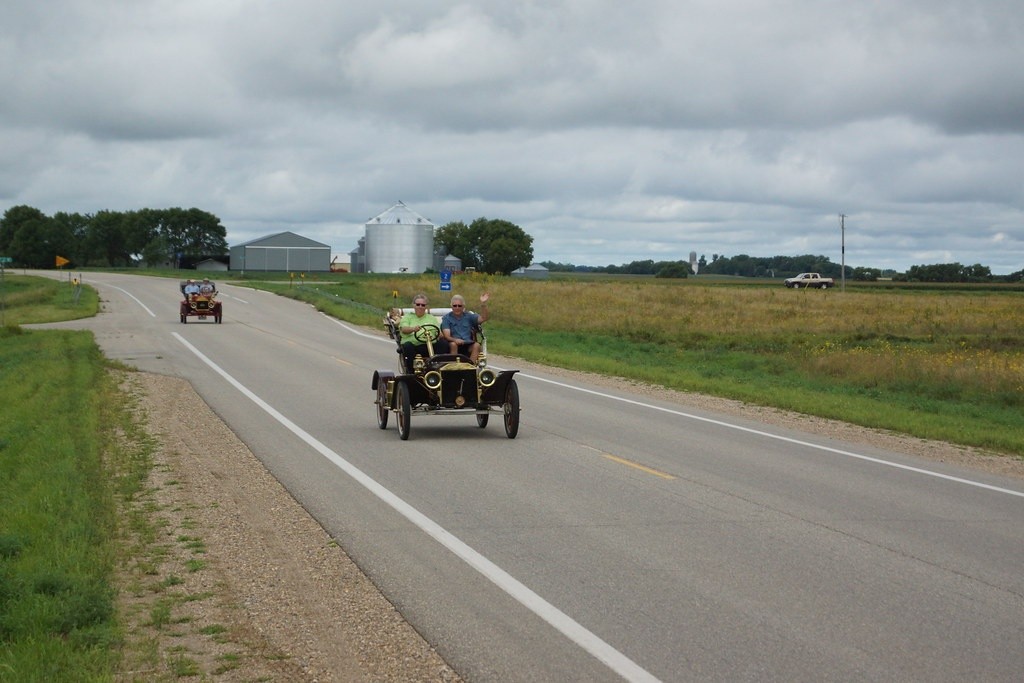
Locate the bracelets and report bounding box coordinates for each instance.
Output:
[480,304,486,307]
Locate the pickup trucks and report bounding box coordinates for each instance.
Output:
[784,273,834,290]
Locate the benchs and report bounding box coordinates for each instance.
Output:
[386,307,487,368]
[180,281,215,301]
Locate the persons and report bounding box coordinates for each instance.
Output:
[398,294,445,376]
[185,279,199,303]
[200,277,213,297]
[440,291,491,365]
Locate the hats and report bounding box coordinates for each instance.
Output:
[190,279,195,283]
[203,277,209,281]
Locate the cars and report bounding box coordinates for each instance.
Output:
[179,280,222,324]
[371,307,521,439]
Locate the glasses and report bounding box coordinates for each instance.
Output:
[416,303,426,307]
[453,305,463,307]
